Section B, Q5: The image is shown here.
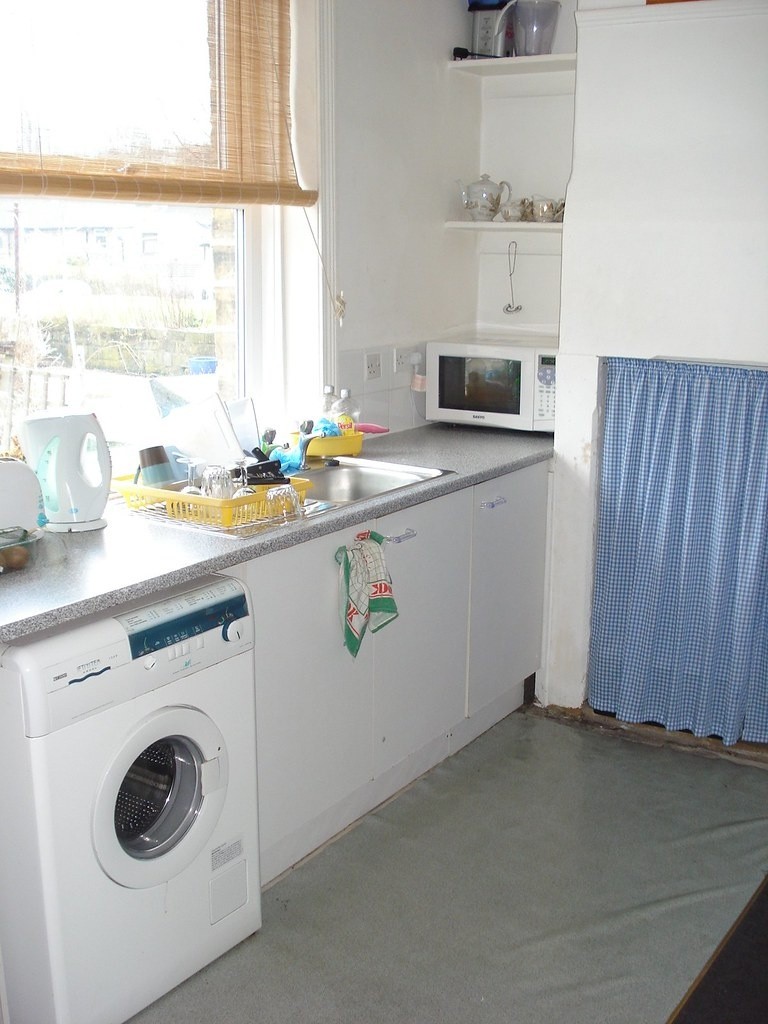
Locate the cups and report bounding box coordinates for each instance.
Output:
[134,445,176,490]
[265,484,301,526]
[165,446,188,481]
[501,198,530,222]
[202,464,232,500]
[533,198,565,223]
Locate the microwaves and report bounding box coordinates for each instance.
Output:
[425,330,558,432]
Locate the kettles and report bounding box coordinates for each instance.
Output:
[16,413,113,533]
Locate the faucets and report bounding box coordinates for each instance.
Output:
[262,429,289,459]
[298,420,326,471]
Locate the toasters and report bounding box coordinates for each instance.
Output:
[0,457,48,546]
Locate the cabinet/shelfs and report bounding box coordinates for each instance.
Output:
[443,53,577,233]
[218,460,552,896]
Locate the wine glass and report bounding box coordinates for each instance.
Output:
[176,457,204,497]
[232,457,256,499]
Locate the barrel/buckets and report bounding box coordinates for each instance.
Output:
[188,357,217,373]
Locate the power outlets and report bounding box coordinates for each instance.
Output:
[394,345,407,373]
[364,351,382,381]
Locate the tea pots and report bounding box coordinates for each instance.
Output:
[456,174,512,221]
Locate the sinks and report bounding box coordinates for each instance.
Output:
[284,456,458,506]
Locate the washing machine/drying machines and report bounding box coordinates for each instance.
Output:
[4,573,262,1022]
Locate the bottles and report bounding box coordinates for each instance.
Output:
[513,0,562,56]
[322,385,339,418]
[331,388,360,436]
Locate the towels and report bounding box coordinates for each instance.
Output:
[339,529,399,658]
